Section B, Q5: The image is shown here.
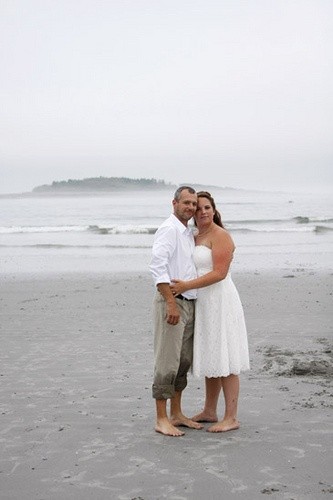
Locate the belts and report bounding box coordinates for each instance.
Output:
[173,293,194,301]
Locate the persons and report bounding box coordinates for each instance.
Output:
[152,186,205,437]
[168,191,249,433]
